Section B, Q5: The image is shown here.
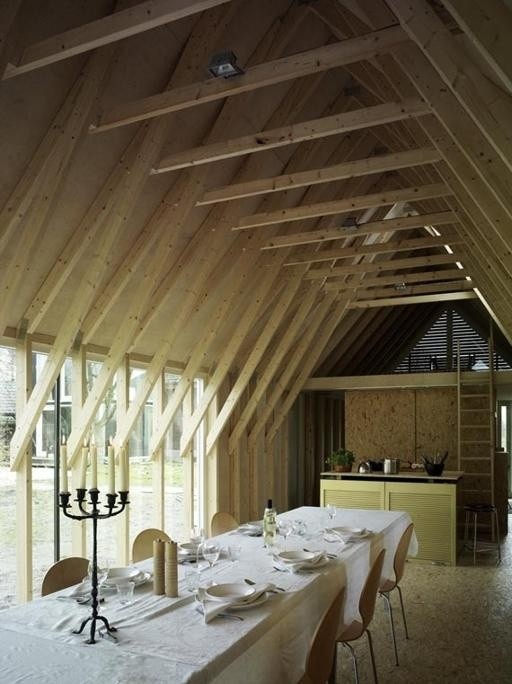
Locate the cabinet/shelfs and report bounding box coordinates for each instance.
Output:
[319,478,461,567]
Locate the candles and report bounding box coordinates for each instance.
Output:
[58,434,130,496]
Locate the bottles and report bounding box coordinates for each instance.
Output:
[262,499,276,546]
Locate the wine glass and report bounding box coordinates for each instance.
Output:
[86,555,110,604]
[188,527,205,569]
[202,542,222,586]
[279,520,293,544]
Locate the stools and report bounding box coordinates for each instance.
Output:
[462,503,502,563]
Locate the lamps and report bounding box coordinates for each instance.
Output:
[206,51,244,80]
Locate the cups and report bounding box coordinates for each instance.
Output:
[228,544,242,562]
[185,571,200,591]
[116,582,135,603]
[295,520,305,535]
[327,504,337,520]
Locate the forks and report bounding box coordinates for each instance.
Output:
[274,567,313,575]
[324,539,355,544]
[194,608,243,622]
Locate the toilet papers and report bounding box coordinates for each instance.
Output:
[153,540,179,598]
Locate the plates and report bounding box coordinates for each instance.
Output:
[83,567,150,589]
[239,522,279,530]
[324,525,371,539]
[195,584,267,610]
[177,543,219,558]
[273,551,329,568]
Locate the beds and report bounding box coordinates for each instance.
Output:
[296,521,415,682]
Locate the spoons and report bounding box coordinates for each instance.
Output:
[54,598,91,604]
[303,548,337,558]
[244,578,286,591]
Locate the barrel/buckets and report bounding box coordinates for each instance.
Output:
[384,457,399,473]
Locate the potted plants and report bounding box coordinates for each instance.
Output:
[325,447,356,473]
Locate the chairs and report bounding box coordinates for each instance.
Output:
[130,528,172,564]
[40,556,101,598]
[209,510,241,538]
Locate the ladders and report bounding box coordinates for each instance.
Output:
[457,337,496,563]
